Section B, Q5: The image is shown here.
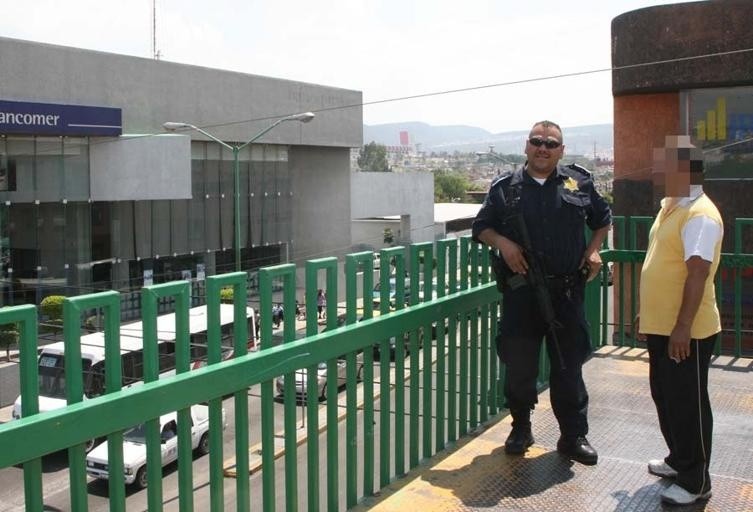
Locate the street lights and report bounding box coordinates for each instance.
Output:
[164,111,314,269]
[476,151,518,172]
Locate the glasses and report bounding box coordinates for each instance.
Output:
[528,137,560,148]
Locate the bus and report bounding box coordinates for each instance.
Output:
[8,300,257,461]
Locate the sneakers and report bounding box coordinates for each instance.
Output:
[649,460,677,476]
[661,484,711,505]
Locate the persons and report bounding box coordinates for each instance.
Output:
[470,122,613,465]
[296,300,300,321]
[634,143,725,505]
[316,289,327,318]
[254,307,260,340]
[271,304,283,328]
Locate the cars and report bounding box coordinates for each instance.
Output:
[86,405,226,489]
[320,249,500,359]
[274,357,364,402]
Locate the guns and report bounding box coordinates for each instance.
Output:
[502,213,568,373]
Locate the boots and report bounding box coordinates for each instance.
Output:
[557,434,596,463]
[504,409,534,454]
